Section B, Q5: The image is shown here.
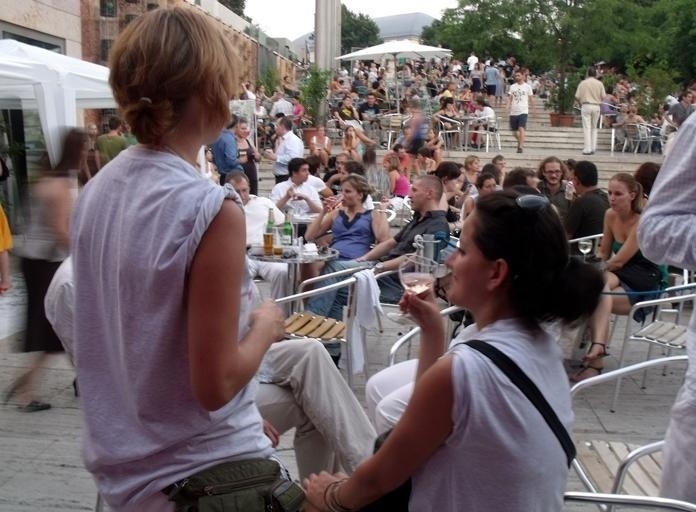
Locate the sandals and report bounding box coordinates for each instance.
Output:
[581,341,611,364]
[20,399,52,413]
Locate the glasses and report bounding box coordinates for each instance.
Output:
[516,184,551,215]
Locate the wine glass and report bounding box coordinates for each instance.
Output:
[386,255,439,326]
[578,239,593,265]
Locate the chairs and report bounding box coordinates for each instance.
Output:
[272,264,468,369]
[568,229,696,413]
[598,102,620,129]
[565,353,696,512]
[374,193,415,223]
[622,122,664,154]
[464,114,503,153]
[434,112,464,152]
[386,113,404,151]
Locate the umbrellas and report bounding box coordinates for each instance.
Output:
[333,39,452,114]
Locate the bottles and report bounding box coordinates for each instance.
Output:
[281,209,293,245]
[326,194,345,212]
[266,208,275,233]
[565,181,573,201]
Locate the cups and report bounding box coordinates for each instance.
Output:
[290,222,298,246]
[262,234,273,255]
[273,227,284,255]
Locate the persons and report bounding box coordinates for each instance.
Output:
[17,127,90,412]
[0,3,287,512]
[197,50,696,512]
[81,115,138,186]
[0,204,14,295]
[40,247,384,485]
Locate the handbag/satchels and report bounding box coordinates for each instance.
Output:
[373,425,412,511]
[166,457,305,512]
[571,366,604,383]
